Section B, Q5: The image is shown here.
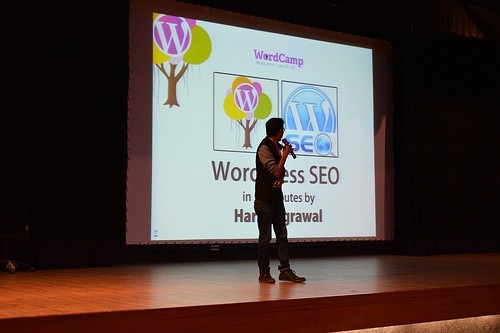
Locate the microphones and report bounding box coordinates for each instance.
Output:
[282,138,296,159]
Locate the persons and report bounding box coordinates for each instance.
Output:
[254,116,308,284]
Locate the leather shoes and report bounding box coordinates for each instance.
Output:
[259,272,275,283]
[279,270,306,283]
